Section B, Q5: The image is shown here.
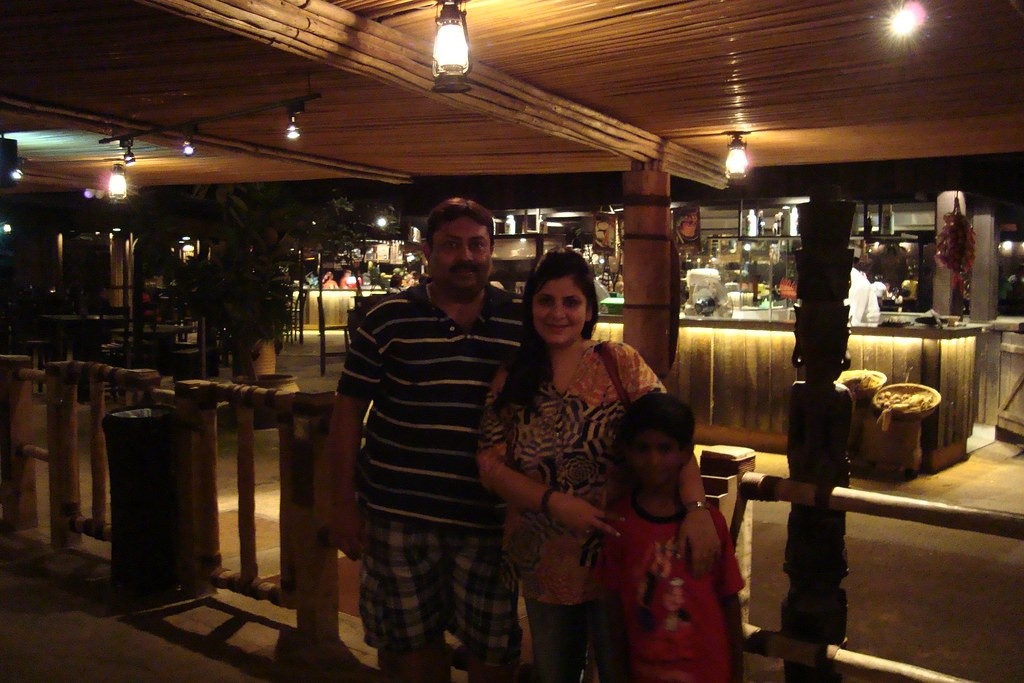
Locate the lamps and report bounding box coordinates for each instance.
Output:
[180,125,201,156]
[286,103,315,140]
[109,133,136,200]
[724,131,750,187]
[432,0,474,95]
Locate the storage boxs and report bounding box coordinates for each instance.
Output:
[600,296,625,314]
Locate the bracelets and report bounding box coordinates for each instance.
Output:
[541,488,557,518]
[684,501,709,513]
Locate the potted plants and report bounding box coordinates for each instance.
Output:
[157,223,299,429]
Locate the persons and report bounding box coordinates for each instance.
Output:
[81,283,128,366]
[477,251,723,683]
[327,198,529,683]
[844,266,918,327]
[591,393,745,683]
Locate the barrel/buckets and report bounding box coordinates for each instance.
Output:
[250,372,301,430]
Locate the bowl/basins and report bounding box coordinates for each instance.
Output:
[839,370,887,403]
[873,383,942,420]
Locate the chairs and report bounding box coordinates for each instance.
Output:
[318,297,351,377]
[283,290,306,344]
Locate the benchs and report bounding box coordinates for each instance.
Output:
[28,337,217,381]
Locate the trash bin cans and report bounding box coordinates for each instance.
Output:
[101,404,185,604]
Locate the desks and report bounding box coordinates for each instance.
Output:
[108,326,194,374]
[38,315,125,381]
